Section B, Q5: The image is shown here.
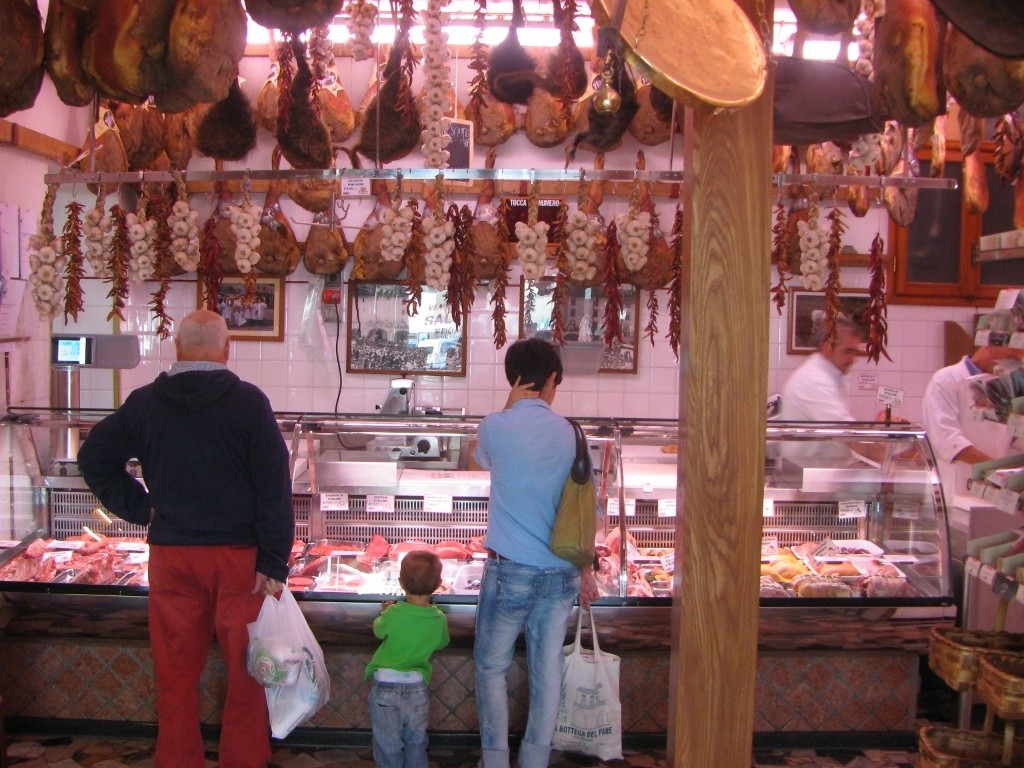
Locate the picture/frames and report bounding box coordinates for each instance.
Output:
[785,283,876,359]
[517,273,642,376]
[342,277,469,379]
[195,270,286,344]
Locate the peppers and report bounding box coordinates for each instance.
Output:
[55,0,899,368]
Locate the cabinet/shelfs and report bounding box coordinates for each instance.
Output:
[1,405,963,758]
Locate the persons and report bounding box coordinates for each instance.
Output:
[922,325,1024,503]
[78,309,295,768]
[363,551,450,768]
[778,318,901,469]
[474,338,599,767]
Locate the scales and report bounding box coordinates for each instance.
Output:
[40,331,146,479]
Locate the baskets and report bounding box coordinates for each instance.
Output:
[918,599,1024,767]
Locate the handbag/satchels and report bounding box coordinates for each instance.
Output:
[248,584,331,740]
[548,418,597,566]
[550,604,626,763]
